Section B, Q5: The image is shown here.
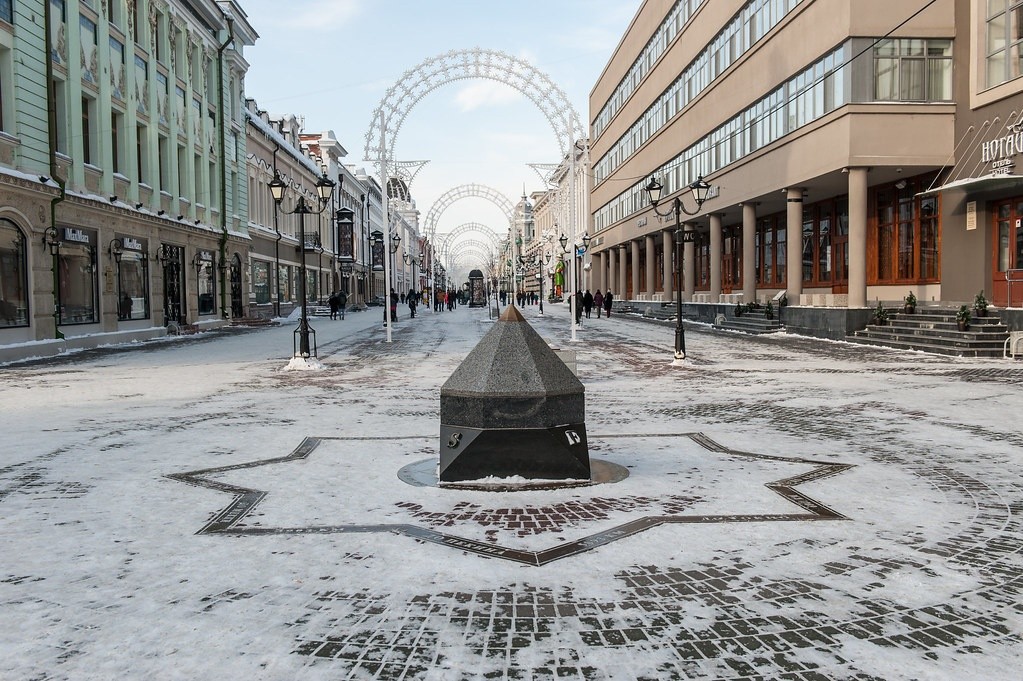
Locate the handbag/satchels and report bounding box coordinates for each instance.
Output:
[593,302,596,310]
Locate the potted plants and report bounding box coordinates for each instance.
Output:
[780,294,788,307]
[903,291,918,314]
[972,289,990,317]
[955,304,972,331]
[734,300,743,317]
[872,301,889,326]
[745,298,760,313]
[763,299,775,320]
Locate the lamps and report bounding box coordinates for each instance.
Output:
[41,226,60,257]
[192,252,203,273]
[155,246,168,268]
[217,256,228,274]
[108,238,124,263]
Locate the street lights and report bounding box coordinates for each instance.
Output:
[643,174,712,360]
[558,232,593,341]
[516,263,530,309]
[420,263,432,309]
[403,251,424,316]
[530,252,551,314]
[368,232,401,343]
[267,168,338,361]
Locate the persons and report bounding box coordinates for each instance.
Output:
[583,289,594,320]
[406,288,429,319]
[400,291,406,304]
[500,289,507,306]
[533,293,539,305]
[337,289,347,320]
[121,291,133,319]
[527,292,530,305]
[390,287,399,312]
[2,297,18,326]
[430,289,463,312]
[594,289,604,318]
[516,290,522,306]
[567,289,584,325]
[603,288,613,318]
[328,290,338,320]
[521,289,526,308]
[553,256,564,298]
[530,290,535,304]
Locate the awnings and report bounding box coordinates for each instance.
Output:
[915,167,1023,198]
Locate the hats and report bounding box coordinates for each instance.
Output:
[607,288,611,293]
